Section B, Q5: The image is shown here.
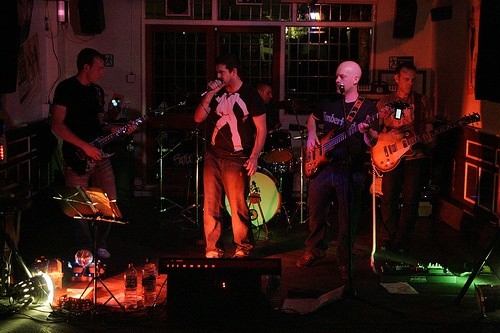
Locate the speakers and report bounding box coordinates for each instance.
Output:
[78,0,106,34]
[159,256,282,314]
[393,0,417,39]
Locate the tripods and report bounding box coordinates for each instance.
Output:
[143,132,204,231]
[53,185,129,321]
[313,93,413,321]
[286,99,311,233]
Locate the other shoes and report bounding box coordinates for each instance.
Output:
[399,248,408,255]
[378,246,389,254]
[296,254,322,267]
[206,250,223,258]
[98,248,110,258]
[233,249,254,259]
[338,259,351,272]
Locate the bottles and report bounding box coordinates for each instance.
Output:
[142,258,157,306]
[124,264,137,309]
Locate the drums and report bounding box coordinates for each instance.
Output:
[224,165,281,226]
[260,129,292,165]
[259,154,298,174]
[291,157,308,197]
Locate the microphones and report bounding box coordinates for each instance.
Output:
[201,80,222,96]
[339,84,345,93]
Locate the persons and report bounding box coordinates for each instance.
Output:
[258,83,273,103]
[194,50,266,258]
[51,48,138,203]
[296,61,380,273]
[376,62,434,245]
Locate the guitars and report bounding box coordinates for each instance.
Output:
[61,113,149,176]
[303,99,411,178]
[371,111,481,172]
